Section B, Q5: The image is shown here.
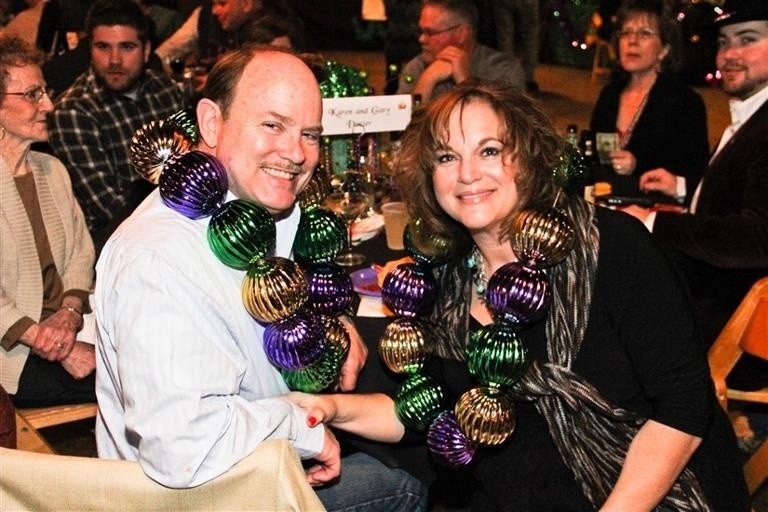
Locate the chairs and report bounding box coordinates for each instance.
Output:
[0,383,96,456]
[0,439,326,512]
[706,275,768,497]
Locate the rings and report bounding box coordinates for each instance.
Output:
[616,165,621,170]
[55,342,63,348]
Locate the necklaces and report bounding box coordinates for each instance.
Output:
[378,139,586,466]
[130,110,355,394]
[467,245,489,307]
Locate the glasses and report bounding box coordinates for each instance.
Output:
[616,28,661,39]
[0,88,54,103]
[416,24,461,37]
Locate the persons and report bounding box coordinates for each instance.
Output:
[95,41,428,512]
[1,0,312,411]
[278,77,753,510]
[395,1,767,441]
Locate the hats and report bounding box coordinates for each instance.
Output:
[714,0,768,27]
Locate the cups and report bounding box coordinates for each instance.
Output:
[380,201,413,250]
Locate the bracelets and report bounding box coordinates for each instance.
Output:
[57,306,83,317]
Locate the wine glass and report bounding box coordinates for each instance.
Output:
[326,191,372,266]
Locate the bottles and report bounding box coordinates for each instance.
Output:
[563,124,581,149]
